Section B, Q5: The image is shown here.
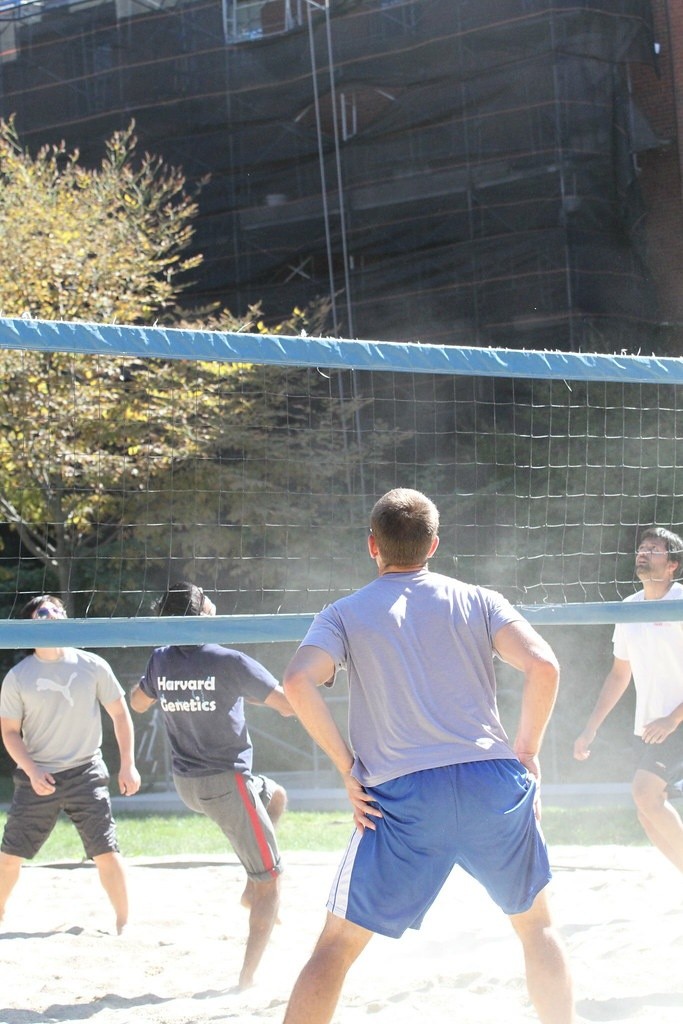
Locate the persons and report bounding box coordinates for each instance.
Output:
[572,529,683,872]
[128,581,295,987]
[281,487,572,1024]
[0,593,141,937]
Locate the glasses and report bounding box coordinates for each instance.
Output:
[197,586,207,616]
[35,606,66,622]
